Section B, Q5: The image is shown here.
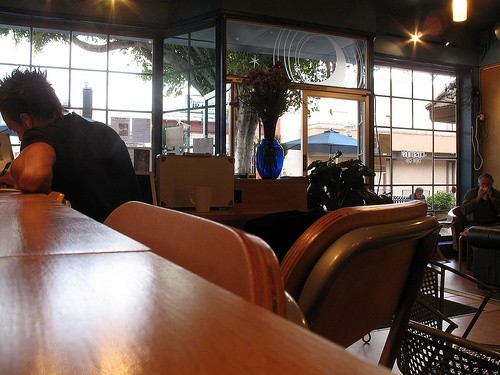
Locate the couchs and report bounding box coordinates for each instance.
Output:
[466,225,500,291]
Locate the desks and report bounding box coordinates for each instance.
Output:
[0,251,393,374]
[0,191,154,256]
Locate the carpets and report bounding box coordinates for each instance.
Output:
[374,296,485,329]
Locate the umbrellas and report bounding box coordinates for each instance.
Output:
[280,129,360,167]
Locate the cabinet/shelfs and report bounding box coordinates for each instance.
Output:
[181,175,308,224]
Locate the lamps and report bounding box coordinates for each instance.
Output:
[452,0,467,22]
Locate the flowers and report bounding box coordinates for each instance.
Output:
[230,60,300,138]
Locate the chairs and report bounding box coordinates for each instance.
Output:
[106,199,500,375]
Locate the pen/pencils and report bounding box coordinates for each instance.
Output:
[0,162,12,176]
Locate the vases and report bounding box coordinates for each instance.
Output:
[257,138,285,179]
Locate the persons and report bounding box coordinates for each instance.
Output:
[461,173,500,225]
[0,67,152,225]
[405,187,425,202]
